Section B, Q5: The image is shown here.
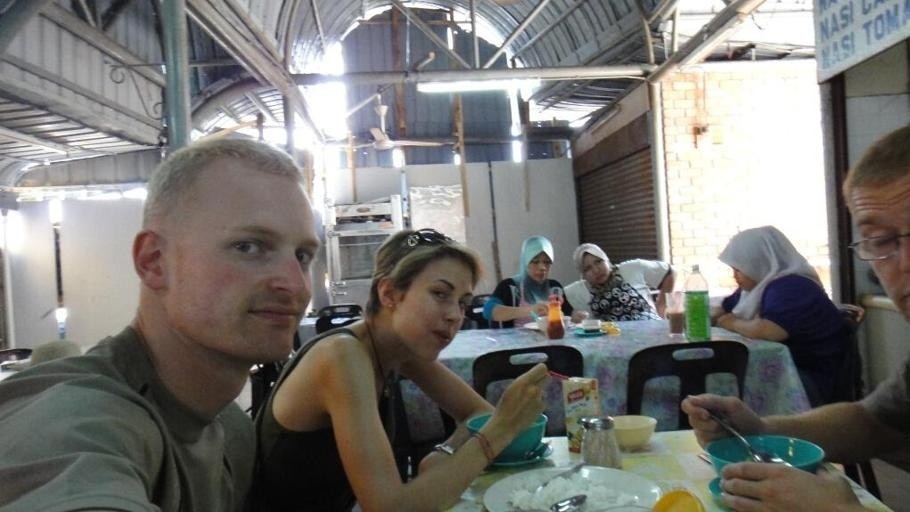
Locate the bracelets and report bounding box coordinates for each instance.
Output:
[434,443,458,454]
[474,431,496,463]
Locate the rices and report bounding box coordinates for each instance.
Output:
[505,470,640,512]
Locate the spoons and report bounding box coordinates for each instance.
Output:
[550,494,587,512]
[707,411,793,466]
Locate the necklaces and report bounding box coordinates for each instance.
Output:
[365,318,393,397]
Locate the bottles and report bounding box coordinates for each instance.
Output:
[684,264,711,345]
[576,416,623,470]
[548,301,565,339]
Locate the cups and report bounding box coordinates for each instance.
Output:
[581,319,601,332]
[666,291,685,337]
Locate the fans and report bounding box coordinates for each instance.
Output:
[336,93,443,151]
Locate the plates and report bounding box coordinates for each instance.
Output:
[482,463,663,512]
[523,322,540,329]
[574,329,607,337]
[709,477,728,499]
[494,441,553,467]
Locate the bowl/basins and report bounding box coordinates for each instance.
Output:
[536,316,572,334]
[706,434,826,480]
[466,412,547,459]
[611,414,658,452]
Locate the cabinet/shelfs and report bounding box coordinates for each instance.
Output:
[324,194,404,311]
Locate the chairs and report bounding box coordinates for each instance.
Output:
[835,302,885,504]
[627,341,749,429]
[315,304,364,335]
[472,345,584,400]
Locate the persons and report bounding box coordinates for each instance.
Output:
[253,230,553,510]
[0,135,322,511]
[564,243,678,323]
[681,122,910,511]
[710,227,855,408]
[481,236,589,328]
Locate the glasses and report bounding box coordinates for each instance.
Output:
[402,227,453,248]
[846,231,908,262]
